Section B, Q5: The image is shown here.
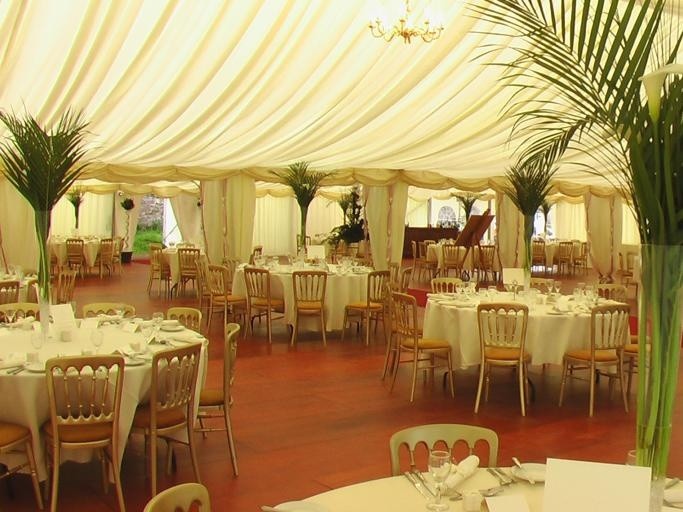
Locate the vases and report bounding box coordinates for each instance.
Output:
[634,241,680,482]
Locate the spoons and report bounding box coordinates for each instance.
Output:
[488,467,516,486]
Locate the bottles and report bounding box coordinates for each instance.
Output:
[430,222,458,228]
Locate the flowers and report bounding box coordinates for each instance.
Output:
[466,0,683,477]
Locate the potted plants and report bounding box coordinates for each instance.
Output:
[279,160,326,245]
[0,98,103,300]
[120,197,135,263]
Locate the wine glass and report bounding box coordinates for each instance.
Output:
[436,238,454,245]
[532,234,568,243]
[422,451,452,510]
[166,237,206,251]
[0,313,197,377]
[479,238,494,246]
[52,228,104,242]
[0,264,26,284]
[252,251,361,273]
[455,278,597,310]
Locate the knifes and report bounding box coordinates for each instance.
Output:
[412,469,438,496]
[512,457,536,485]
[404,472,431,503]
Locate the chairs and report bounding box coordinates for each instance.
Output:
[389,423,500,475]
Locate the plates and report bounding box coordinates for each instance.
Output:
[662,478,683,503]
[273,501,330,512]
[511,462,548,483]
